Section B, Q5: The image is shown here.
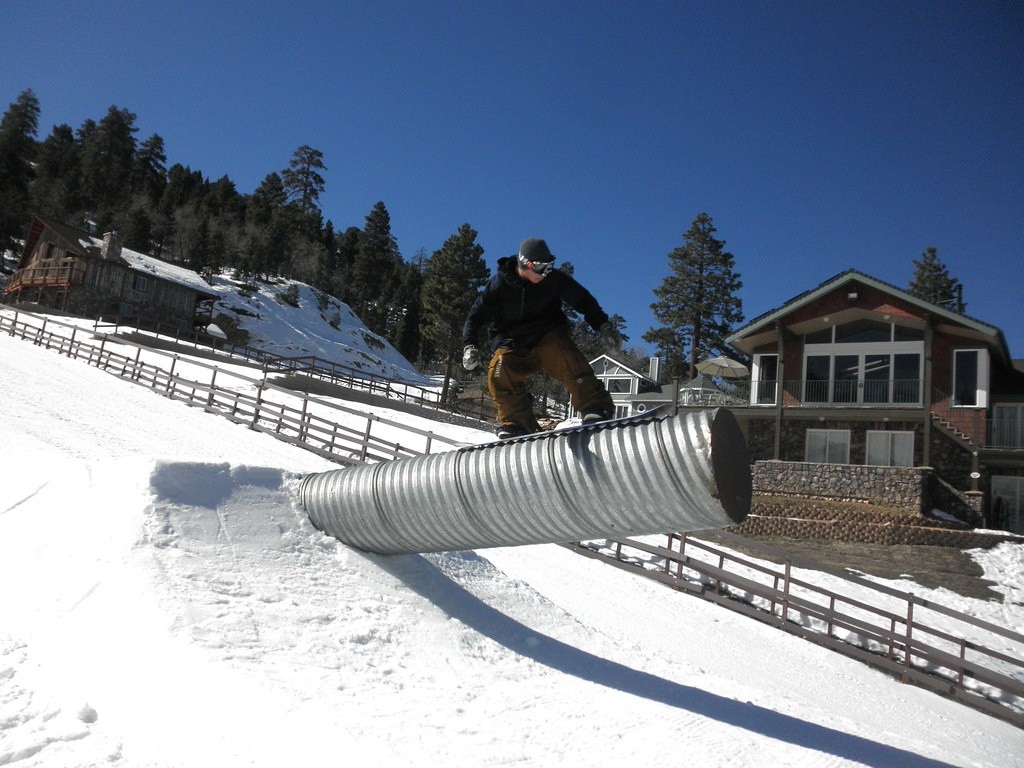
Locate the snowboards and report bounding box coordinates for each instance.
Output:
[453,402,670,452]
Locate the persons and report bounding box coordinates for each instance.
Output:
[463,238,622,439]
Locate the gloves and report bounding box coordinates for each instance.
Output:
[601,322,622,354]
[462,345,480,371]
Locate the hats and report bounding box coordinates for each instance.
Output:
[519,237,556,270]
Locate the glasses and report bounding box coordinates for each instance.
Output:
[532,258,555,275]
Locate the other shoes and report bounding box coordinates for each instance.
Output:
[497,426,526,437]
[582,406,608,422]
